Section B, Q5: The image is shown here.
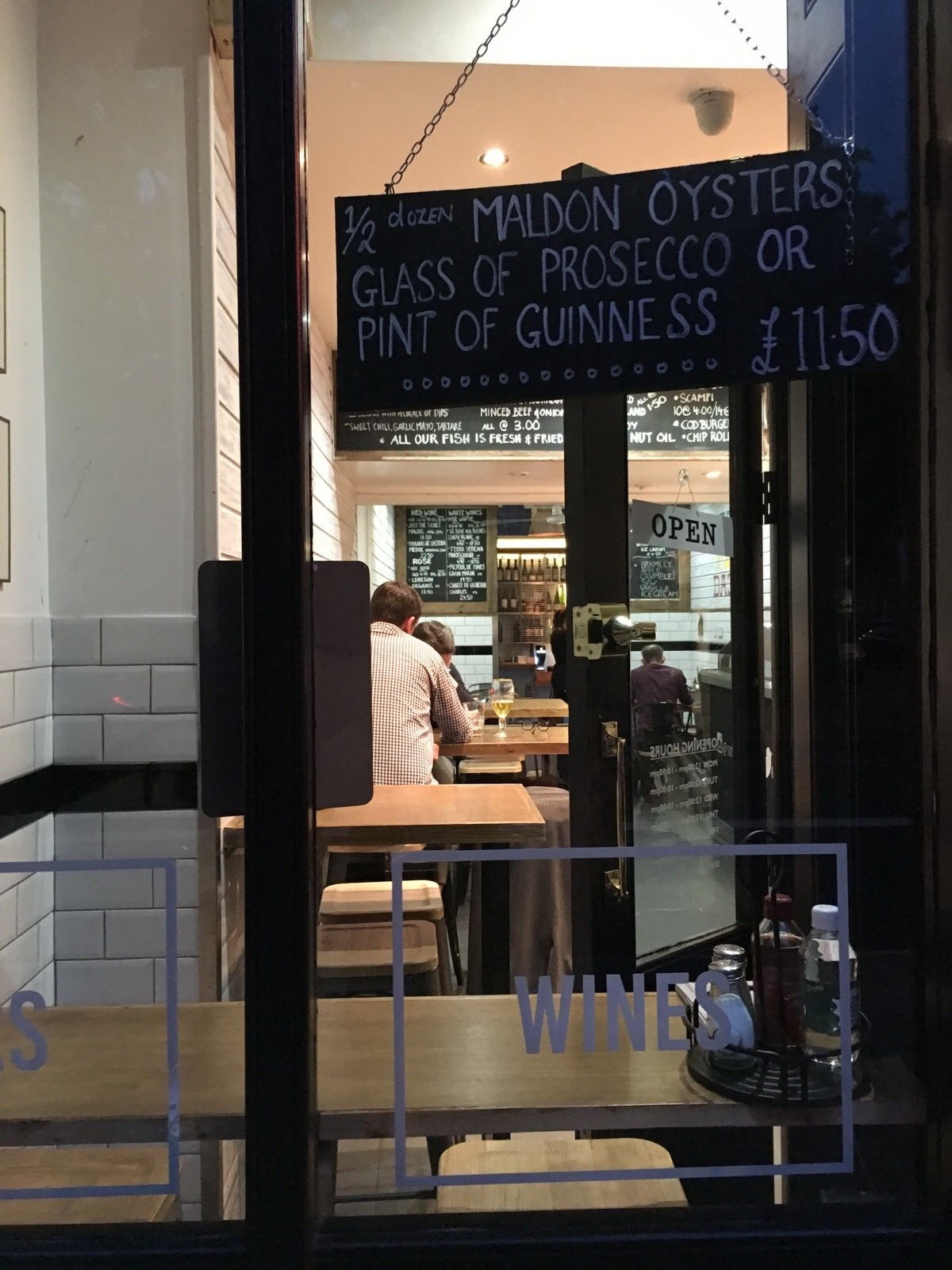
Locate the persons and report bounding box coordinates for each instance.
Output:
[630,644,693,795]
[369,581,473,892]
[413,620,477,727]
[550,609,568,700]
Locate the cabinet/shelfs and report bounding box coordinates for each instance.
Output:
[498,582,564,645]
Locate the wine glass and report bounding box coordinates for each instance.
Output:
[490,678,515,738]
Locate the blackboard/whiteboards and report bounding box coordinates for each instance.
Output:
[333,145,903,412]
[330,349,730,461]
[394,505,497,615]
[628,503,691,611]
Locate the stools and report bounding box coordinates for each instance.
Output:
[315,753,687,1211]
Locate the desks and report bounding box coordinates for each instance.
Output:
[0,993,924,1147]
[485,698,569,717]
[437,725,568,755]
[224,783,546,846]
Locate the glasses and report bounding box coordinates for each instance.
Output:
[518,719,549,734]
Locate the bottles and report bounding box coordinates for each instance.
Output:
[522,599,545,611]
[498,621,502,642]
[797,904,861,1066]
[750,893,805,1049]
[501,589,517,609]
[515,617,552,642]
[498,558,566,582]
[708,944,755,1029]
[546,585,565,611]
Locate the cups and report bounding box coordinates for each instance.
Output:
[462,701,484,736]
